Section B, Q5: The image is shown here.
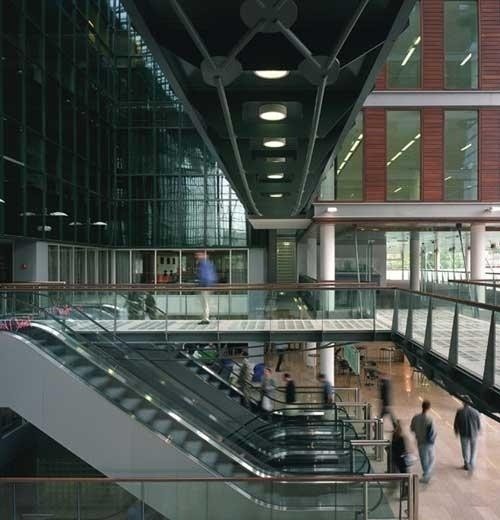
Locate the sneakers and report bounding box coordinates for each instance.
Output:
[464,464,475,471]
[197,319,210,324]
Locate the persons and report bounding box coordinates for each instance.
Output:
[388,418,409,475]
[367,361,388,379]
[193,345,204,361]
[237,351,254,408]
[142,290,157,320]
[282,373,299,417]
[275,345,288,373]
[360,356,377,368]
[379,375,391,419]
[123,286,145,319]
[318,372,335,425]
[195,250,220,325]
[204,343,217,359]
[453,392,481,474]
[260,366,277,418]
[335,347,355,375]
[410,400,437,484]
